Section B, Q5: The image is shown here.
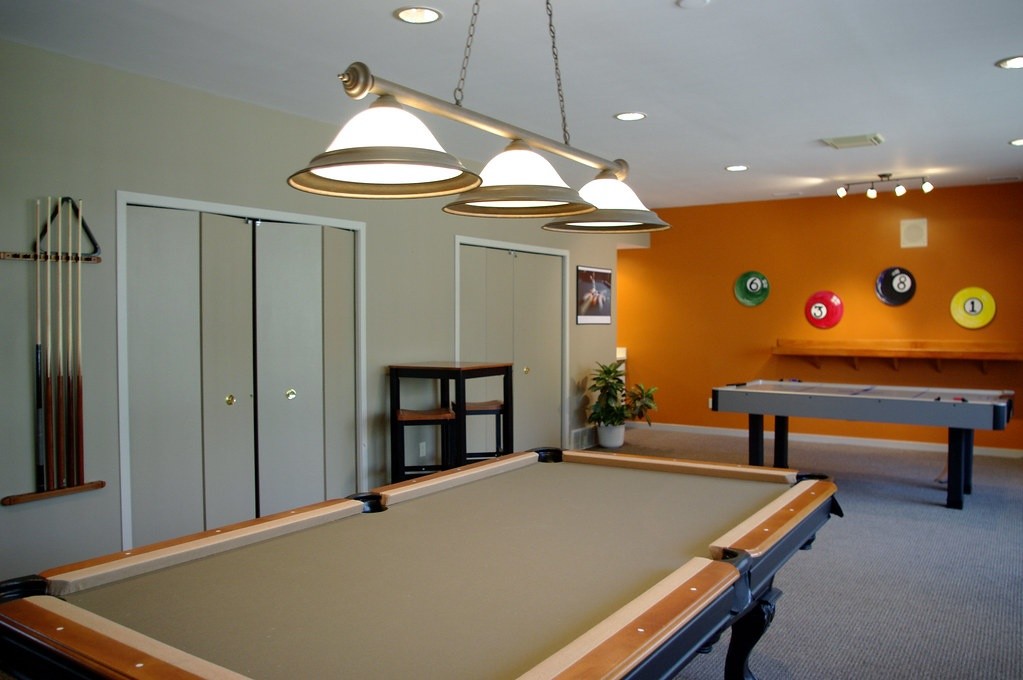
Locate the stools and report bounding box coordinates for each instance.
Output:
[397,409,454,482]
[451,400,503,461]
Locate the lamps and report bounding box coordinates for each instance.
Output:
[286,0,672,234]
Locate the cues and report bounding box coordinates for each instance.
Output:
[30,192,88,495]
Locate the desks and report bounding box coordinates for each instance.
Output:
[11,445,844,680]
[710,380,1015,511]
[388,360,514,485]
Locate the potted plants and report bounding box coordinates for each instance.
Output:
[584,361,659,448]
